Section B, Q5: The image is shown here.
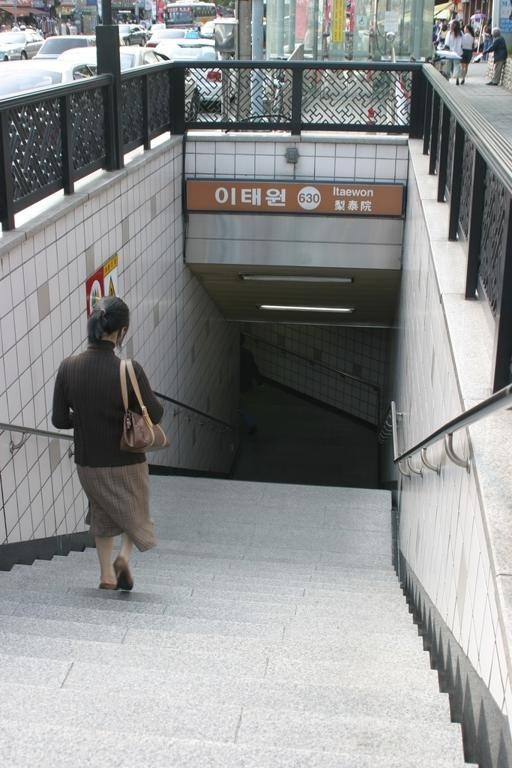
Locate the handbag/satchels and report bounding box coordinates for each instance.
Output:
[121,409,170,452]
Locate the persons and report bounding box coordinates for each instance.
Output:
[433,17,508,86]
[240,332,265,434]
[51,296,165,592]
[1,12,77,40]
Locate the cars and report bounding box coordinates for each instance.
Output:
[117,14,239,55]
[32,34,97,60]
[56,47,200,123]
[0,29,45,61]
[0,58,97,98]
[155,38,237,108]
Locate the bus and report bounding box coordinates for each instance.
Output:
[161,0,218,30]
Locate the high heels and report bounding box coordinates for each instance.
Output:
[99,555,135,590]
